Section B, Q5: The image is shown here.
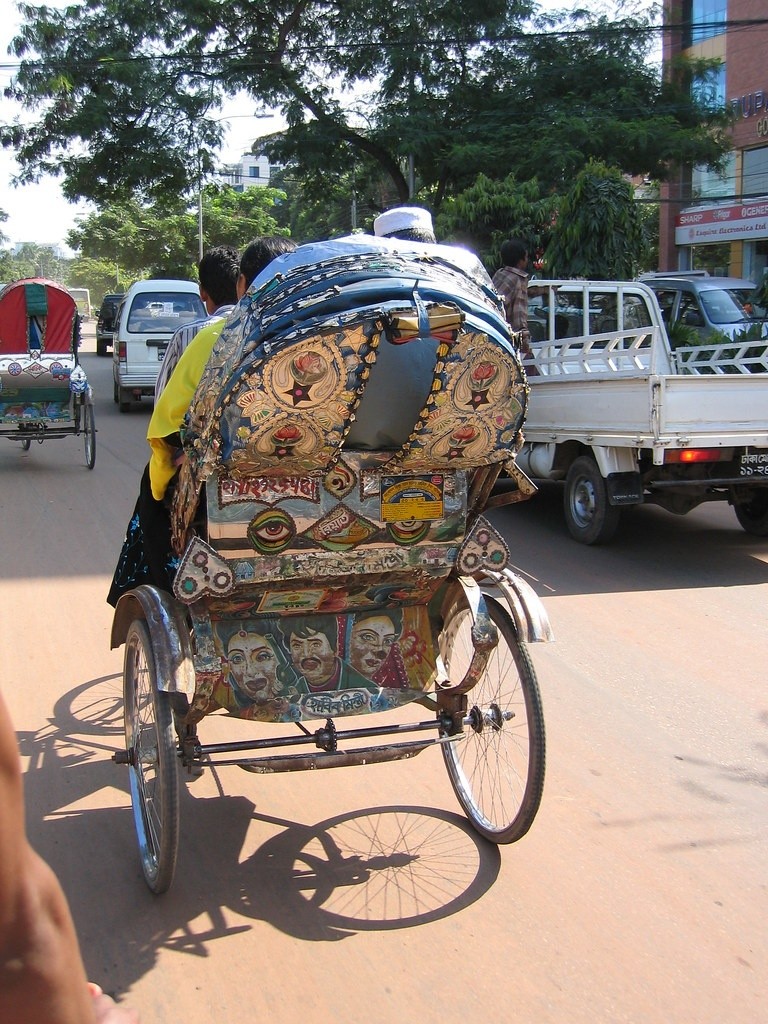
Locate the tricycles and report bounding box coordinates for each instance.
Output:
[105,206,548,896]
[1,277,98,471]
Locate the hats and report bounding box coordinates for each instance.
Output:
[373,206,434,237]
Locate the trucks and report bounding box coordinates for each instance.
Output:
[500,278,765,544]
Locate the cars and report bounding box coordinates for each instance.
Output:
[639,270,709,277]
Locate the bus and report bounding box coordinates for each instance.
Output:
[67,288,91,323]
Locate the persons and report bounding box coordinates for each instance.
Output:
[492,242,540,376]
[0,689,142,1024]
[154,245,243,409]
[106,236,299,608]
[374,207,437,244]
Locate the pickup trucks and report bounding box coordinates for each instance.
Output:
[95,294,124,355]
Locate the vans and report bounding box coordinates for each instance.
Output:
[104,279,210,412]
[640,278,768,349]
